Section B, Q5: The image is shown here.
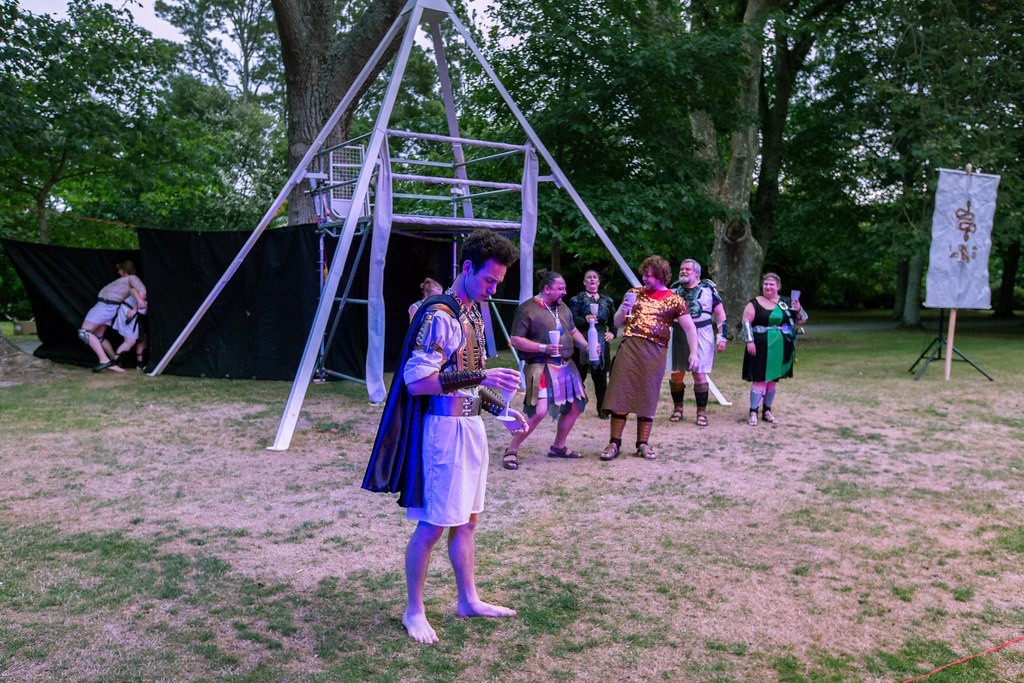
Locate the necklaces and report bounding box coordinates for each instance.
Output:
[444,287,487,368]
[587,290,598,297]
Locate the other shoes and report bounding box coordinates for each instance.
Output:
[110,362,125,373]
[93,360,114,372]
[137,365,146,372]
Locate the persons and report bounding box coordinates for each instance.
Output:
[503,268,599,470]
[78,258,148,373]
[670,258,728,425]
[408,278,443,323]
[570,270,618,419]
[600,255,699,461]
[742,273,808,425]
[360,228,530,644]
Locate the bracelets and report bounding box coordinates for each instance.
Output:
[716,333,727,344]
[539,344,547,353]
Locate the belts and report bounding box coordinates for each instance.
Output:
[122,301,133,310]
[428,395,482,416]
[99,297,122,305]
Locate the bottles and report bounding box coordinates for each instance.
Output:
[588,319,600,361]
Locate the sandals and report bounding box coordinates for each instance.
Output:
[601,443,620,458]
[763,412,777,423]
[748,412,758,426]
[697,414,708,425]
[548,446,583,457]
[670,410,683,421]
[637,445,656,458]
[503,448,519,468]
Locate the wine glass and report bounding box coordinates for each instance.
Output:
[496,377,520,421]
[625,293,635,317]
[788,290,801,310]
[549,331,562,357]
[590,303,599,323]
